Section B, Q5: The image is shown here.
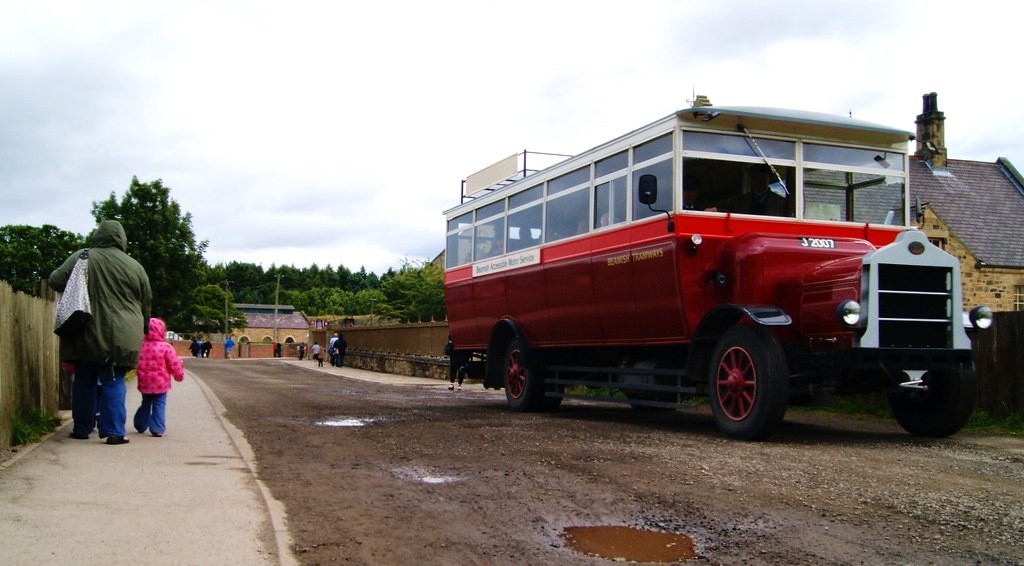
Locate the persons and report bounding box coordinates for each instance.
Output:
[304,343,308,360]
[465,166,788,263]
[273,341,282,357]
[330,333,338,367]
[448,335,466,390]
[333,334,347,368]
[47,219,153,446]
[298,342,305,360]
[311,342,319,365]
[224,337,235,360]
[317,347,324,367]
[134,318,184,439]
[188,340,212,358]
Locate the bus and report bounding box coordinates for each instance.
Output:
[442,103,992,438]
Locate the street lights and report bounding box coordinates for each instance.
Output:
[221,279,234,337]
[369,297,377,325]
[274,273,285,342]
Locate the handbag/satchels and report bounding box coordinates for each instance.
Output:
[333,348,339,357]
[53,248,94,340]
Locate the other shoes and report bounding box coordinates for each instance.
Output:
[458,384,462,390]
[69,433,89,439]
[106,437,130,444]
[448,384,454,389]
